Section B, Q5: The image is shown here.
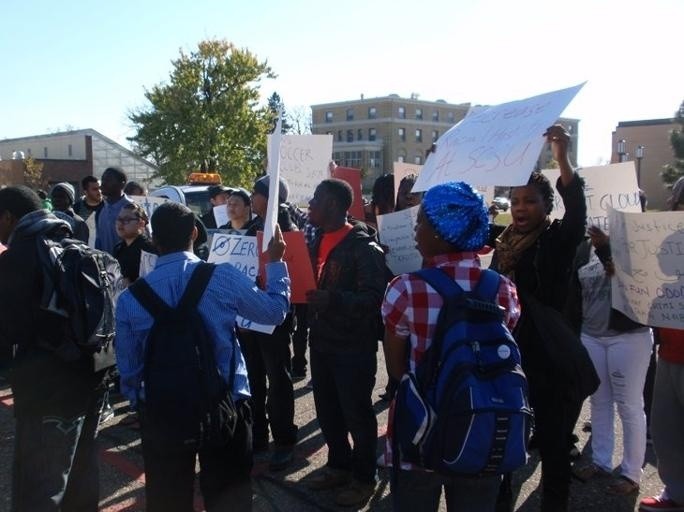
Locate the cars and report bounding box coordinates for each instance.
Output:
[146,172,255,234]
[492,196,510,212]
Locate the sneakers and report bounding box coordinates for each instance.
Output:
[307,471,343,490]
[639,491,677,510]
[564,443,580,459]
[269,448,294,470]
[99,406,114,423]
[291,370,306,380]
[575,463,600,482]
[607,478,636,496]
[335,478,374,508]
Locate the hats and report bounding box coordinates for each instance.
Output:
[422,180,491,250]
[52,181,75,205]
[208,184,233,201]
[255,173,288,204]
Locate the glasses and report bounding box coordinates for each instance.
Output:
[116,217,138,224]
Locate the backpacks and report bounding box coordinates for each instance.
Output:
[394,265,535,482]
[32,231,123,375]
[128,261,238,449]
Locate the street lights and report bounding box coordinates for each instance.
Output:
[616,137,644,192]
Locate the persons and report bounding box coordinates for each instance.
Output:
[1,123,683,511]
